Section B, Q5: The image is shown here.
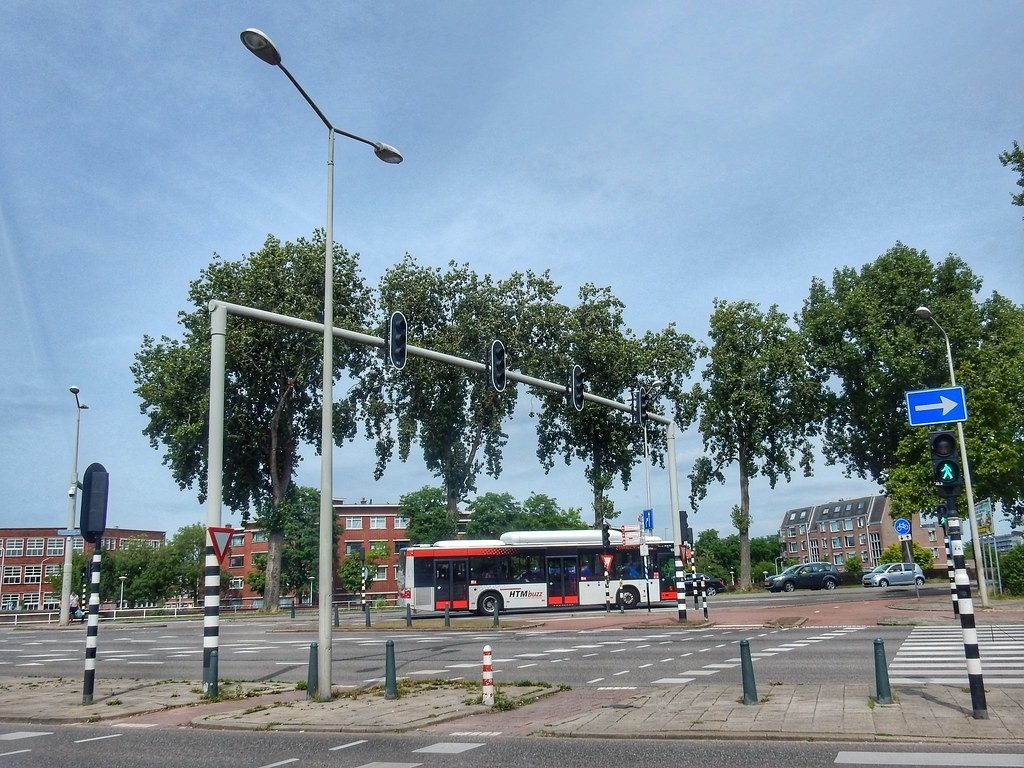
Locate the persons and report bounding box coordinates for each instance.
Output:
[891,566,896,572]
[439,563,642,585]
[70,591,80,623]
[802,569,807,574]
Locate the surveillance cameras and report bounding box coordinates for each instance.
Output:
[68,490,75,497]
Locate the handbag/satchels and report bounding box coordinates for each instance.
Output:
[70,600,73,607]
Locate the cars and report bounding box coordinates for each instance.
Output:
[862,562,925,587]
[685,572,726,597]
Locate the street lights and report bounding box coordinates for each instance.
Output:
[60,384,89,625]
[241,27,404,702]
[917,306,991,610]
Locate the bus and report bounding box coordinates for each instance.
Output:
[399,525,692,616]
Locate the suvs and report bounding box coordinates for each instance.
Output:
[764,561,843,592]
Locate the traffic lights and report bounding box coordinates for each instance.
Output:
[602,525,611,550]
[929,430,963,497]
[638,387,650,429]
[390,311,407,370]
[573,364,587,411]
[937,504,949,526]
[491,340,507,392]
